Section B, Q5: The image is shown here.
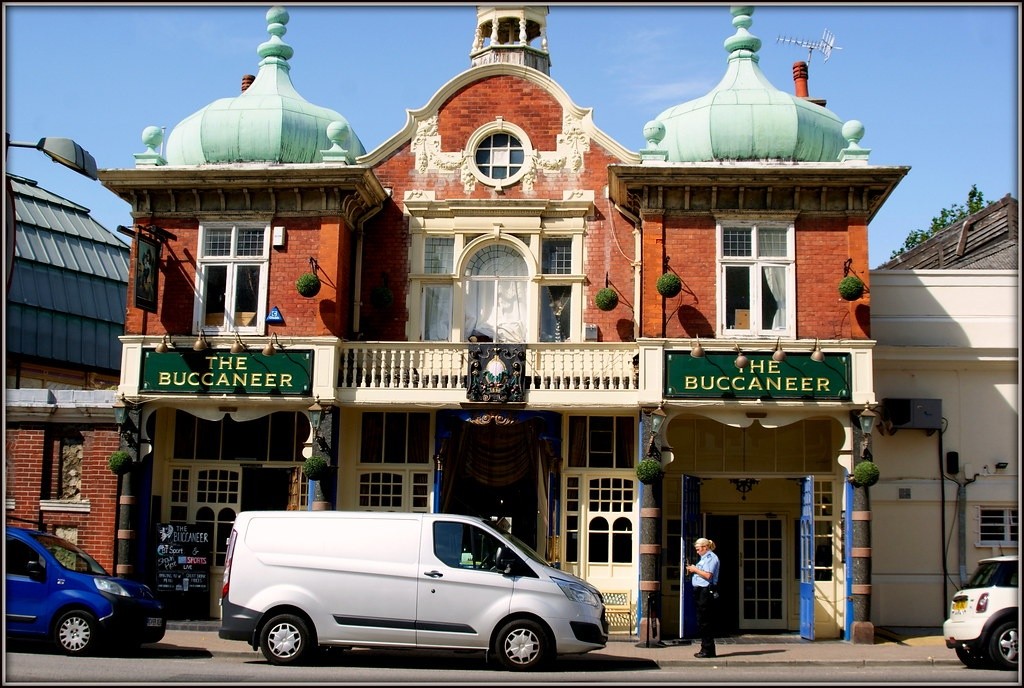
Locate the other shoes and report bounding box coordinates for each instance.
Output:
[694,652,717,658]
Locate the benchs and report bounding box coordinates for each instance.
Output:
[600,587,632,638]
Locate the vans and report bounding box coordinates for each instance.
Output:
[218,509,612,670]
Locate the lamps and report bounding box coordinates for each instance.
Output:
[730,478,761,501]
[690,334,706,359]
[154,332,176,354]
[262,331,282,357]
[230,331,247,354]
[859,399,876,461]
[810,337,825,363]
[193,329,209,351]
[773,335,787,362]
[645,400,667,462]
[113,392,134,447]
[733,344,749,368]
[310,394,326,450]
[995,462,1008,469]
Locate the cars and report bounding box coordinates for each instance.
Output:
[4,525,169,655]
[941,555,1020,668]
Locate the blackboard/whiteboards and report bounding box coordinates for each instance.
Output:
[154,523,213,621]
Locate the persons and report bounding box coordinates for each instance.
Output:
[685,538,720,657]
